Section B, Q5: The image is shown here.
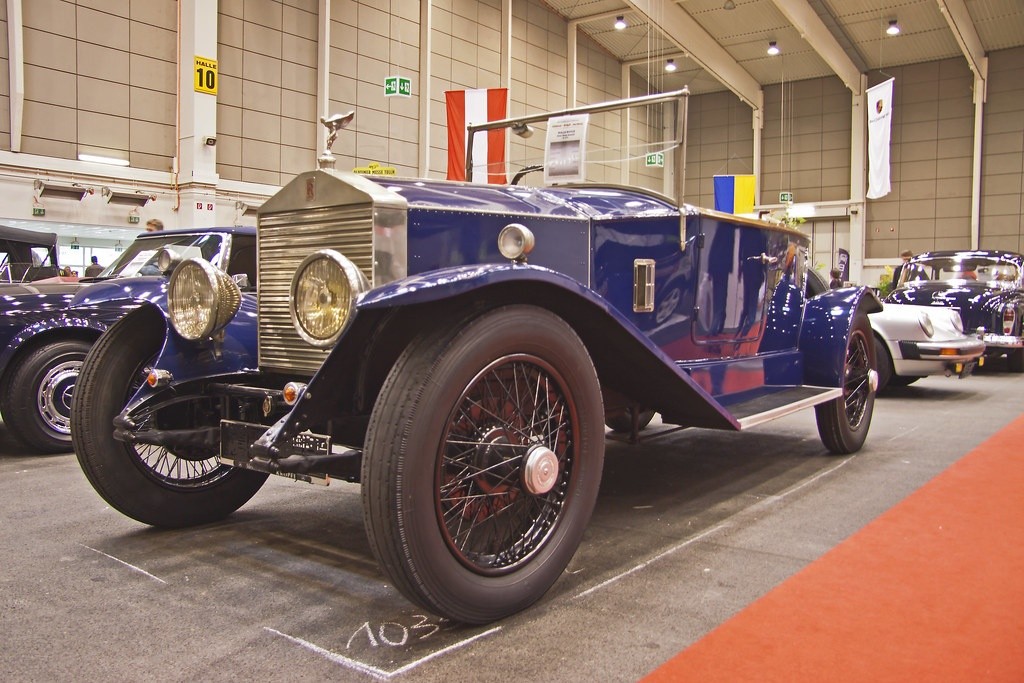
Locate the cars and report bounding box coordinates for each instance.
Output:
[868,301,986,397]
[882,249,1024,372]
[0,222,96,297]
[0,226,259,453]
[65,87,886,629]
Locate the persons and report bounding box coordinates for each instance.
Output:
[85,256,105,278]
[829,267,843,290]
[892,249,930,291]
[139,217,170,276]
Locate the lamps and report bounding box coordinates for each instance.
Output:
[34,180,85,201]
[102,186,150,206]
